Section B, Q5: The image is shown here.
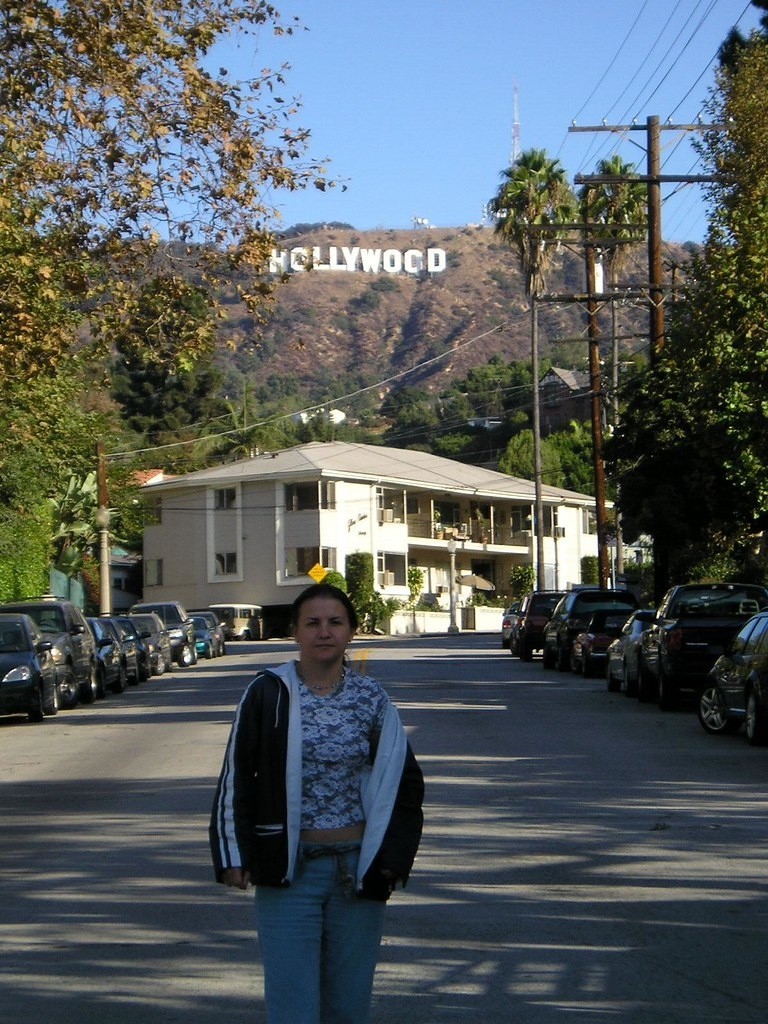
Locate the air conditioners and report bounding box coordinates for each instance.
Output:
[378,509,394,523]
[378,572,394,586]
[436,586,449,593]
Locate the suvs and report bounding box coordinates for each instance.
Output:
[509,588,570,664]
[541,588,640,674]
[0,595,98,710]
[698,604,768,746]
[632,581,768,714]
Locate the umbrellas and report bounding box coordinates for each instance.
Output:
[455,574,495,591]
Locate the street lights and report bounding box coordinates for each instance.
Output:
[446,538,461,635]
[93,502,114,617]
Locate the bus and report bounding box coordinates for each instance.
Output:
[208,603,263,641]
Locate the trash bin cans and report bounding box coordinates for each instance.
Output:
[256,617,269,640]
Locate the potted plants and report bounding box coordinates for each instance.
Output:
[434,526,445,539]
[479,516,488,544]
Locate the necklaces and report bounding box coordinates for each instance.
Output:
[311,673,344,690]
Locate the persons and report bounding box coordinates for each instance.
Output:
[209,583,425,1024]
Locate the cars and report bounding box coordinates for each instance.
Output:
[605,608,659,698]
[569,607,634,678]
[0,613,58,722]
[83,600,226,699]
[501,601,520,648]
